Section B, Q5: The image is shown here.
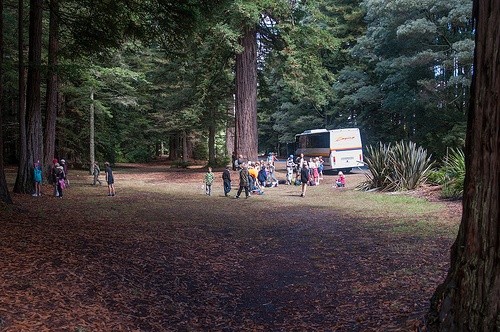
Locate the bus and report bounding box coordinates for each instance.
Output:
[293,128,364,172]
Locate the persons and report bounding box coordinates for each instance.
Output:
[300,161,309,198]
[236,162,250,199]
[222,165,232,197]
[104,162,115,196]
[336,171,346,187]
[91,160,102,186]
[203,167,215,196]
[51,158,70,197]
[32,163,43,197]
[237,152,325,192]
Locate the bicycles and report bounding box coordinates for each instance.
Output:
[240,156,300,196]
